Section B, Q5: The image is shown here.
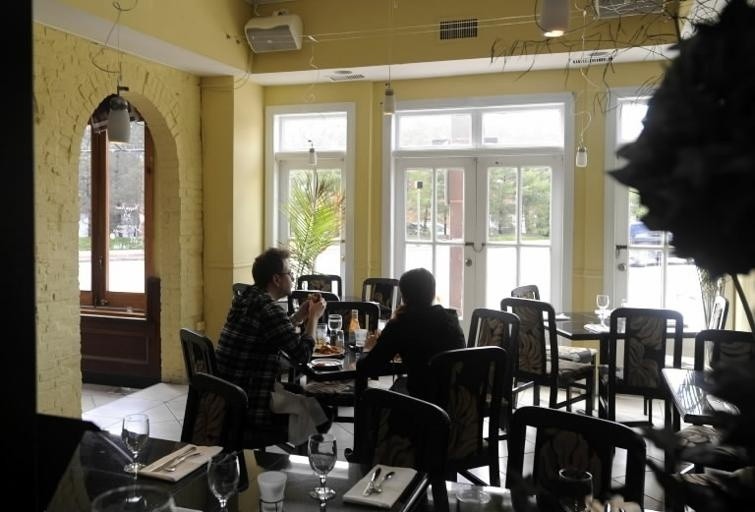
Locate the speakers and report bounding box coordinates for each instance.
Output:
[592,0,666,18]
[244,14,303,54]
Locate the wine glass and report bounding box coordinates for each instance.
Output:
[595,293,611,328]
[121,412,149,473]
[208,453,240,511]
[305,432,337,500]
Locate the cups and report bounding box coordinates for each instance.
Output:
[454,489,494,512]
[353,329,368,346]
[557,468,594,512]
[256,470,286,512]
[314,313,345,345]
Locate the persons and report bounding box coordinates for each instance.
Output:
[217,249,329,457]
[344,265,466,465]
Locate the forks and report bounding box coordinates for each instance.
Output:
[372,470,396,494]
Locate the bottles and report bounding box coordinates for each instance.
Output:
[348,309,360,346]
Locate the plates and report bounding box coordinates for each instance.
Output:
[309,357,343,368]
[310,345,346,357]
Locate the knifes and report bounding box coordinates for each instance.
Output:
[151,445,196,473]
[362,467,381,496]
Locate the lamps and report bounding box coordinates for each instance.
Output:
[89,1,146,144]
[540,1,569,39]
[570,8,593,166]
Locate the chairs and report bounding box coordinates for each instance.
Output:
[175,269,755,512]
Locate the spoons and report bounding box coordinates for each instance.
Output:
[164,450,201,473]
